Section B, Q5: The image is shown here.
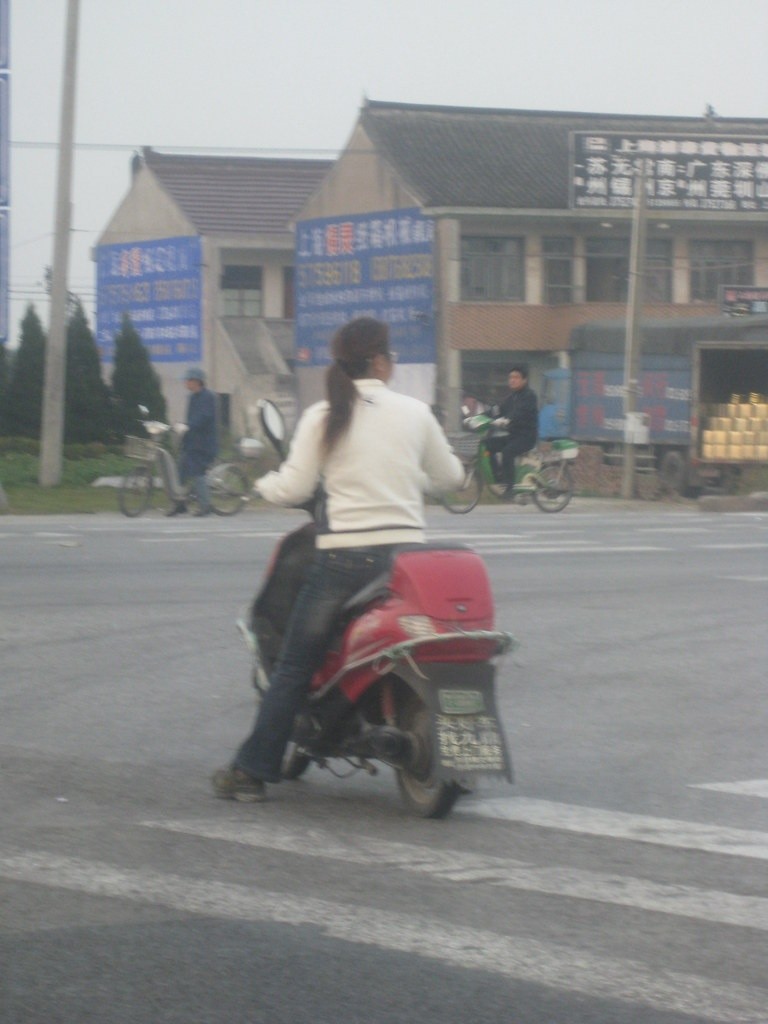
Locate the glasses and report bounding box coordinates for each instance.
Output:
[382,352,398,362]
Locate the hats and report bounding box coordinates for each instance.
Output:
[177,368,205,380]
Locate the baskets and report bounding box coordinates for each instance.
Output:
[448,432,480,457]
[123,434,158,462]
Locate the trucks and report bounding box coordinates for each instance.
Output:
[535,312,768,502]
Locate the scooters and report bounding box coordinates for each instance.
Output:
[114,402,253,517]
[439,404,582,514]
[235,394,519,819]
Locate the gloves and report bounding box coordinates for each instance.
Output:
[492,416,511,427]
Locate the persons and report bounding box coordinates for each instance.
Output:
[211,316,465,803]
[165,366,218,520]
[479,366,538,496]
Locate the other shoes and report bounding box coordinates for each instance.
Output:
[503,485,513,496]
[212,771,266,802]
[167,503,186,516]
[193,506,211,515]
